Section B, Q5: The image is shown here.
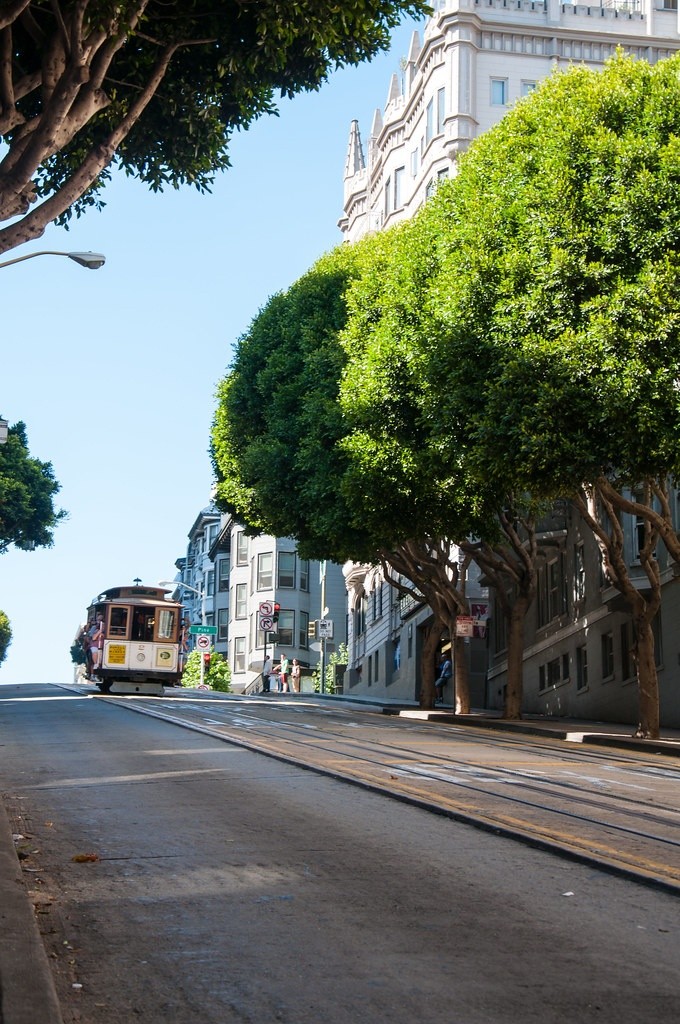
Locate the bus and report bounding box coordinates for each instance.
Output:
[83,578,188,697]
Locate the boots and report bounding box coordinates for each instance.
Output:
[281,683,288,693]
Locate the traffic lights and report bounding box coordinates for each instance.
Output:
[308,622,316,637]
[205,654,210,671]
[269,633,280,642]
[273,602,280,623]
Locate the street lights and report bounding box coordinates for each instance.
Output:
[156,581,206,685]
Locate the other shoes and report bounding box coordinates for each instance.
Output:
[95,678,100,681]
[90,677,95,680]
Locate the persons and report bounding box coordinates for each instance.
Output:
[435,654,451,703]
[83,615,189,687]
[263,653,300,693]
